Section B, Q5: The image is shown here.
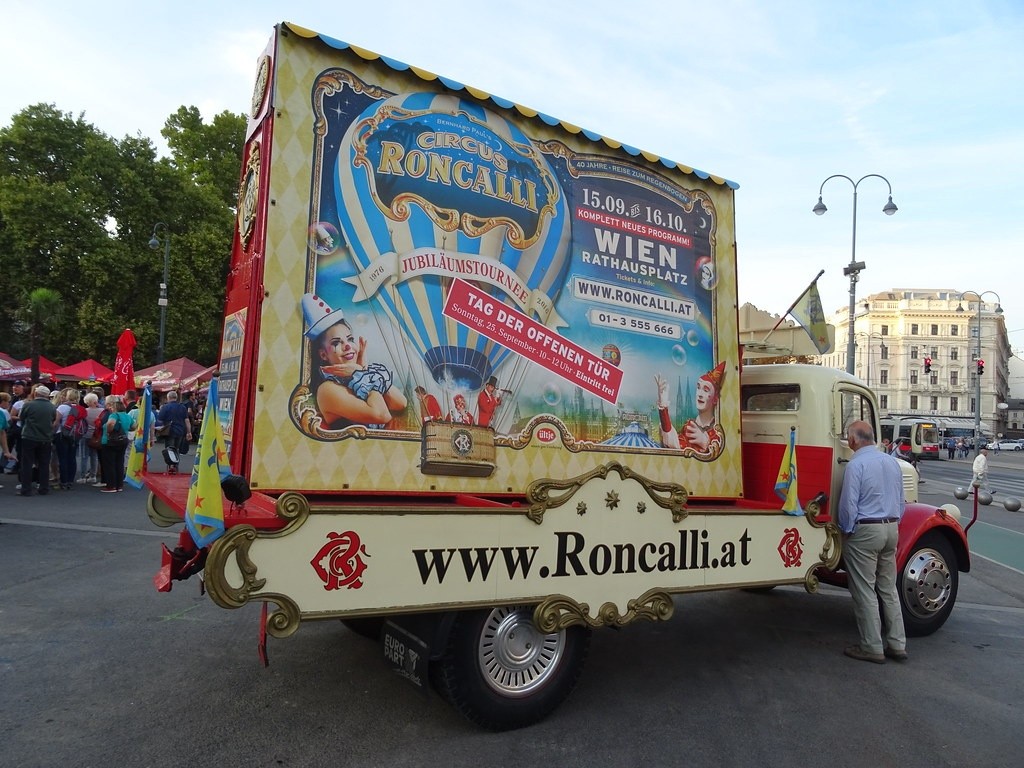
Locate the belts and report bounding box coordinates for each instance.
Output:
[856,518,896,524]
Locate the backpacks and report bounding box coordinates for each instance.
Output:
[60,403,88,443]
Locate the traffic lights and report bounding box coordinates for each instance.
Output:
[977,359,985,375]
[924,357,931,373]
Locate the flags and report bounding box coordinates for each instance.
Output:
[123,386,151,490]
[789,280,831,354]
[774,431,803,516]
[185,380,232,549]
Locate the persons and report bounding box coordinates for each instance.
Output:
[654,361,726,452]
[882,439,926,483]
[948,436,969,460]
[992,439,999,456]
[302,293,407,431]
[0,381,207,496]
[445,394,475,425]
[967,449,997,494]
[415,386,443,426]
[838,421,907,664]
[474,376,502,428]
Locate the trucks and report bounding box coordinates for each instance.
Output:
[135,18,991,736]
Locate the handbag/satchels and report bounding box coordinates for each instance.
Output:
[155,424,170,437]
[89,425,102,448]
[108,412,128,446]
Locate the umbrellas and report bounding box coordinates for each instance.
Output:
[110,328,139,407]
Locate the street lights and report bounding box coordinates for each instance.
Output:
[147,223,169,365]
[955,290,1004,459]
[811,172,899,440]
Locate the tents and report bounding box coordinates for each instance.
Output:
[0,351,217,403]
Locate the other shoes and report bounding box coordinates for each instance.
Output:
[100,486,117,492]
[887,649,907,660]
[846,645,885,664]
[16,490,29,496]
[86,477,96,482]
[92,482,107,487]
[67,481,76,490]
[77,478,86,483]
[60,482,68,490]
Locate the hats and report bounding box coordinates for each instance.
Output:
[12,380,25,387]
[300,294,344,339]
[35,386,51,397]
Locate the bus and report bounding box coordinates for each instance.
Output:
[879,412,940,463]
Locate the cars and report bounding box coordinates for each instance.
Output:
[969,436,1024,452]
